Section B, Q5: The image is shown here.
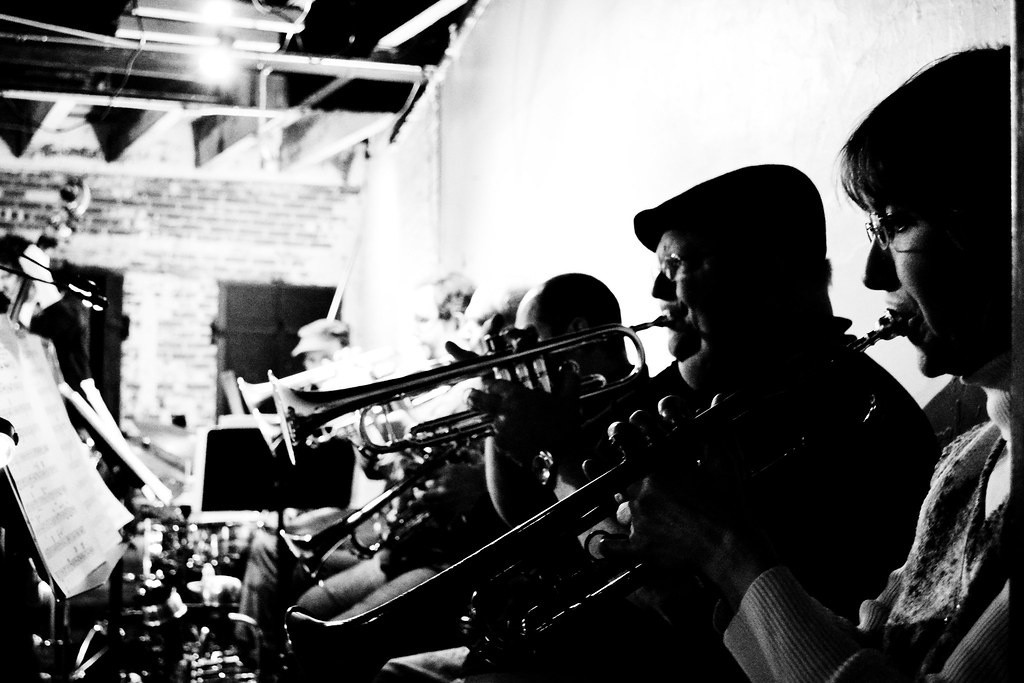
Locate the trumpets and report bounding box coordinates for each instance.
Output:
[235,305,913,683]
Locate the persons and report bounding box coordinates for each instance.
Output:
[0,234,94,441]
[231,43,1012,683]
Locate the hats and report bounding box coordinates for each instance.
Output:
[633,164,827,257]
[291,319,348,356]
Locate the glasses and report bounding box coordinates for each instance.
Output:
[865,214,911,250]
